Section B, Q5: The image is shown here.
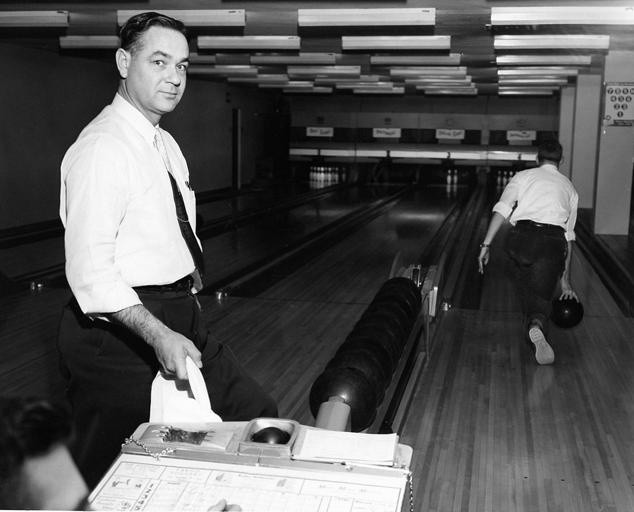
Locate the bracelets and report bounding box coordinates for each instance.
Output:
[480,243,491,248]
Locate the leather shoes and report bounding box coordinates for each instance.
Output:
[528,324,555,365]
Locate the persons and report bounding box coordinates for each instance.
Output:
[0,399,243,512]
[53,13,278,486]
[478,139,579,364]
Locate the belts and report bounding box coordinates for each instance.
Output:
[519,220,562,231]
[138,273,194,292]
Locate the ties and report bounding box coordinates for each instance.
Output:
[154,128,204,280]
